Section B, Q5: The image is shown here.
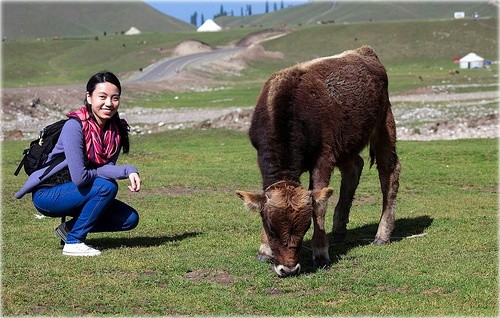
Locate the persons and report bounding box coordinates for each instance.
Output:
[15,70,140,257]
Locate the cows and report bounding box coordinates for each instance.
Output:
[235,44,401,275]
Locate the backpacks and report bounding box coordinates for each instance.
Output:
[13,117,82,181]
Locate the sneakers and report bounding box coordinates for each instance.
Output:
[62,242,102,257]
[55,222,70,242]
